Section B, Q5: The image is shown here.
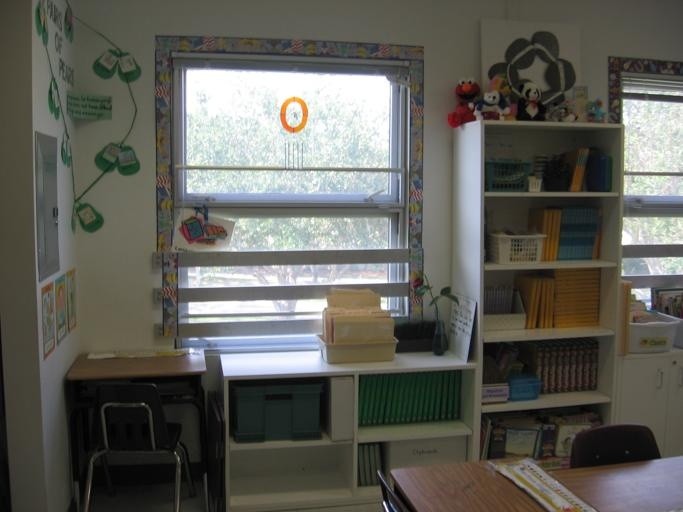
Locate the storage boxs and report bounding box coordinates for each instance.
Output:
[627,311,681,353]
[380,436,467,477]
[229,381,323,442]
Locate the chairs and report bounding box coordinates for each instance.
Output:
[570,423,662,469]
[376,469,410,512]
[84,382,195,512]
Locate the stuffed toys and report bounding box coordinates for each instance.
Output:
[447,78,481,129]
[545,101,579,124]
[514,82,547,121]
[581,97,607,123]
[490,74,517,122]
[473,88,506,122]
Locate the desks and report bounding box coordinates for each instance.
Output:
[390,462,547,512]
[63,349,210,511]
[546,455,683,512]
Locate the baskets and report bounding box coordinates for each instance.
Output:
[484,160,532,193]
[485,223,548,266]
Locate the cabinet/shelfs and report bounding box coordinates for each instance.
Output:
[449,121,624,462]
[614,353,683,458]
[221,350,476,511]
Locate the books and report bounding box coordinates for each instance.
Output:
[524,338,599,394]
[619,279,683,355]
[529,205,602,262]
[358,443,382,487]
[484,267,602,329]
[567,144,591,193]
[359,369,462,426]
[481,410,603,461]
